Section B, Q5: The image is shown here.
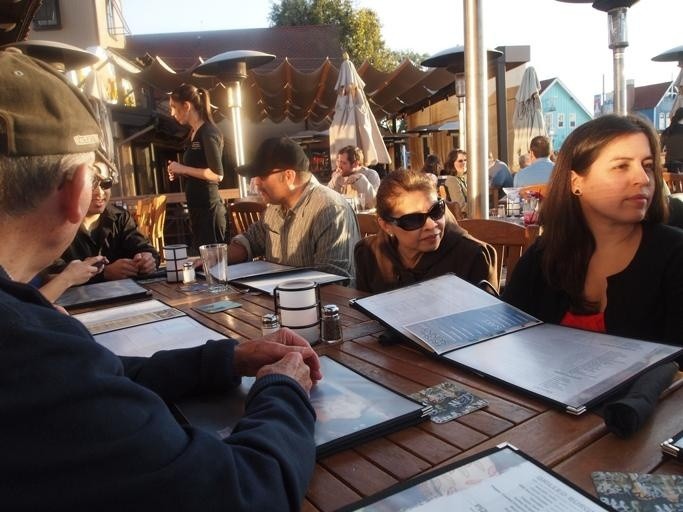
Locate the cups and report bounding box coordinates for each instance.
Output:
[199,243,229,293]
[524,198,539,225]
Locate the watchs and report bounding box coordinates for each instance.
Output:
[165,79,230,256]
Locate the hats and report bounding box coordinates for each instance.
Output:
[234,137,310,177]
[0,46,119,172]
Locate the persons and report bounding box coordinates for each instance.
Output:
[42,154,161,284]
[329,145,380,214]
[0,43,327,512]
[420,102,683,374]
[350,166,499,299]
[196,138,362,291]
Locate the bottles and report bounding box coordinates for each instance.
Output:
[259,314,280,336]
[182,262,196,284]
[497,204,505,218]
[320,304,345,345]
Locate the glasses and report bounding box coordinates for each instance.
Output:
[381,197,445,230]
[93,178,112,191]
[260,169,285,181]
[454,160,467,163]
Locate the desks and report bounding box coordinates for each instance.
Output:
[18,251,683,512]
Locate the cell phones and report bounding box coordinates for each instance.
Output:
[92,256,109,267]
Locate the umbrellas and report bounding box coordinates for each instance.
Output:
[325,48,390,176]
[512,67,543,163]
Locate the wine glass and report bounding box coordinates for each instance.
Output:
[502,188,519,219]
[350,192,366,214]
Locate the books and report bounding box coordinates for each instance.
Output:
[179,351,434,461]
[334,443,625,512]
[218,255,351,292]
[46,270,226,365]
[349,276,681,417]
